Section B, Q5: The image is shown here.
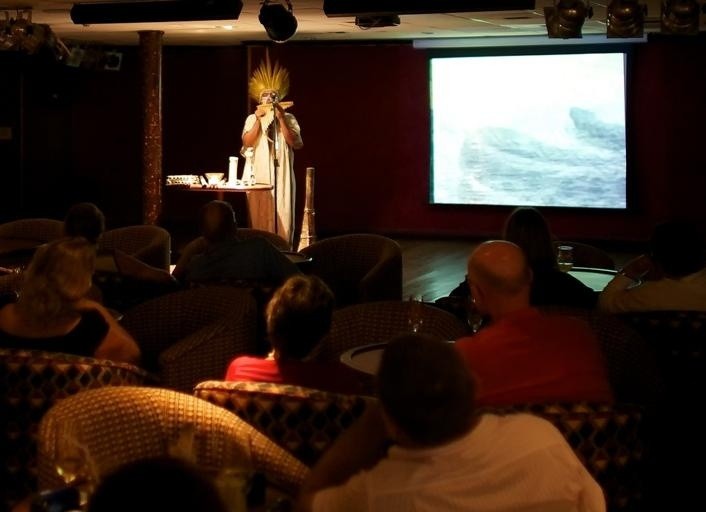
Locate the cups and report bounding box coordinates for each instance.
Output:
[557,244,576,275]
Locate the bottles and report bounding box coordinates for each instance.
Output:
[228,156,239,184]
[242,147,257,186]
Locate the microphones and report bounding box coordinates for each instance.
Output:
[270,93,277,101]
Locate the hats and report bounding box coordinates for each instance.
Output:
[248,48,290,103]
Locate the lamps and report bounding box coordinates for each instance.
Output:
[543,0,592,41]
[603,1,647,37]
[659,1,705,38]
[256,0,300,43]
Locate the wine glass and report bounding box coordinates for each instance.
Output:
[465,296,483,335]
[52,418,96,506]
[406,291,427,334]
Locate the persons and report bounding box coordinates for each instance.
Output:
[0,420,48,510]
[448,238,613,403]
[0,235,144,362]
[83,451,229,512]
[238,86,307,254]
[433,203,599,316]
[590,221,705,315]
[60,200,171,278]
[293,327,610,512]
[222,271,367,395]
[171,198,286,290]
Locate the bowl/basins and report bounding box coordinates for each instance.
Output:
[167,174,199,185]
[205,172,225,184]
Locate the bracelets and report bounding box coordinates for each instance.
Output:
[619,268,634,280]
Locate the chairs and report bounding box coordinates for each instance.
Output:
[92,220,169,275]
[171,230,291,284]
[327,298,459,355]
[36,387,322,512]
[607,302,703,465]
[301,233,405,302]
[2,349,145,492]
[472,398,643,512]
[116,288,260,396]
[192,380,384,489]
[2,219,65,273]
